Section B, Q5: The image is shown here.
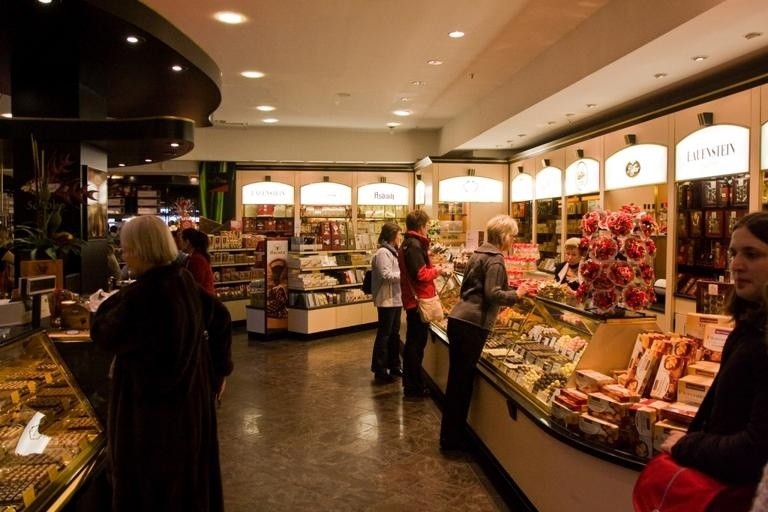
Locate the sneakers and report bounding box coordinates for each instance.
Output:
[372,365,432,403]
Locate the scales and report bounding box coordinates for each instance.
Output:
[0,274,57,329]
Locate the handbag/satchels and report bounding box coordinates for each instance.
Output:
[629,450,734,512]
[361,270,371,295]
[415,294,445,324]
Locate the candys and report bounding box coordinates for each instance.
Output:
[438,306,589,405]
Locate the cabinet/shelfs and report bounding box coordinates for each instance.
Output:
[511,171,768,328]
[2,174,468,336]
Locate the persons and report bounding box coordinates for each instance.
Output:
[438,213,534,449]
[397,208,445,401]
[106,215,131,291]
[553,237,582,293]
[369,222,404,386]
[167,218,201,251]
[178,228,214,292]
[659,210,768,511]
[88,212,236,512]
